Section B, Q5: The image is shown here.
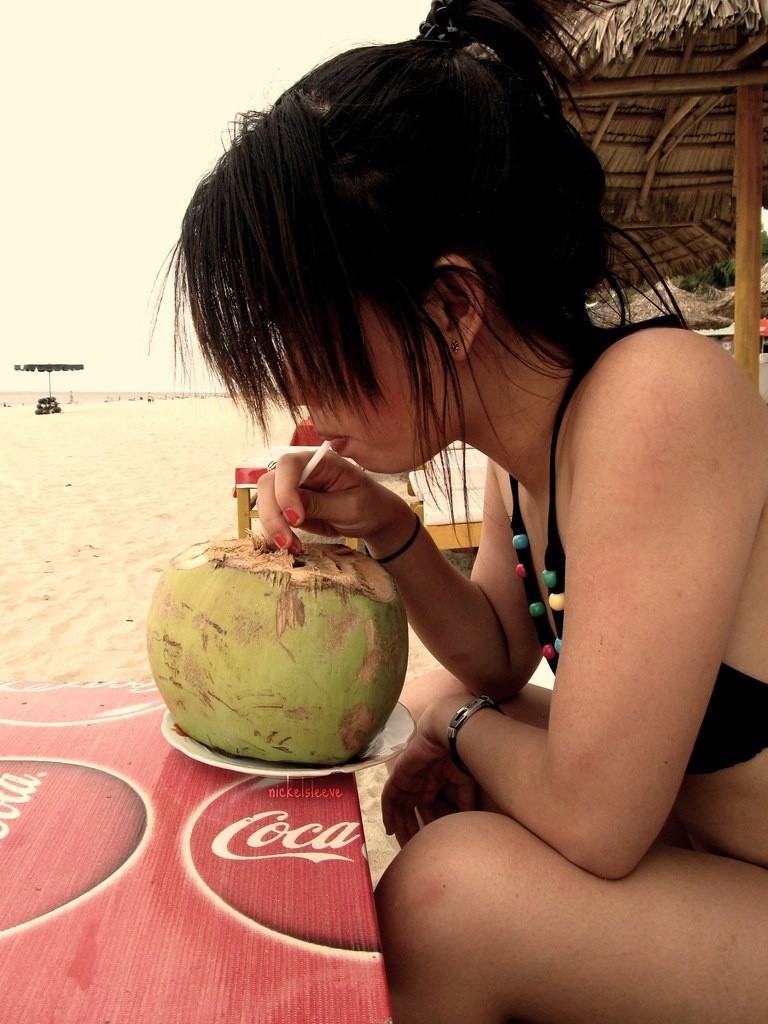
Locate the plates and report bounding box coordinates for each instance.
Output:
[162,702,416,780]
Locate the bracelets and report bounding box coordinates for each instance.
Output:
[364,512,420,562]
[447,695,503,775]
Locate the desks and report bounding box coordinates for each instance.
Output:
[0,681,396,1024]
[233,446,359,551]
[289,417,326,445]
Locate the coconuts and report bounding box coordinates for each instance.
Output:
[146,532,409,766]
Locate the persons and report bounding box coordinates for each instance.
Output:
[173,2,768,1023]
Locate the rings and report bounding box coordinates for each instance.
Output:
[267,460,277,471]
